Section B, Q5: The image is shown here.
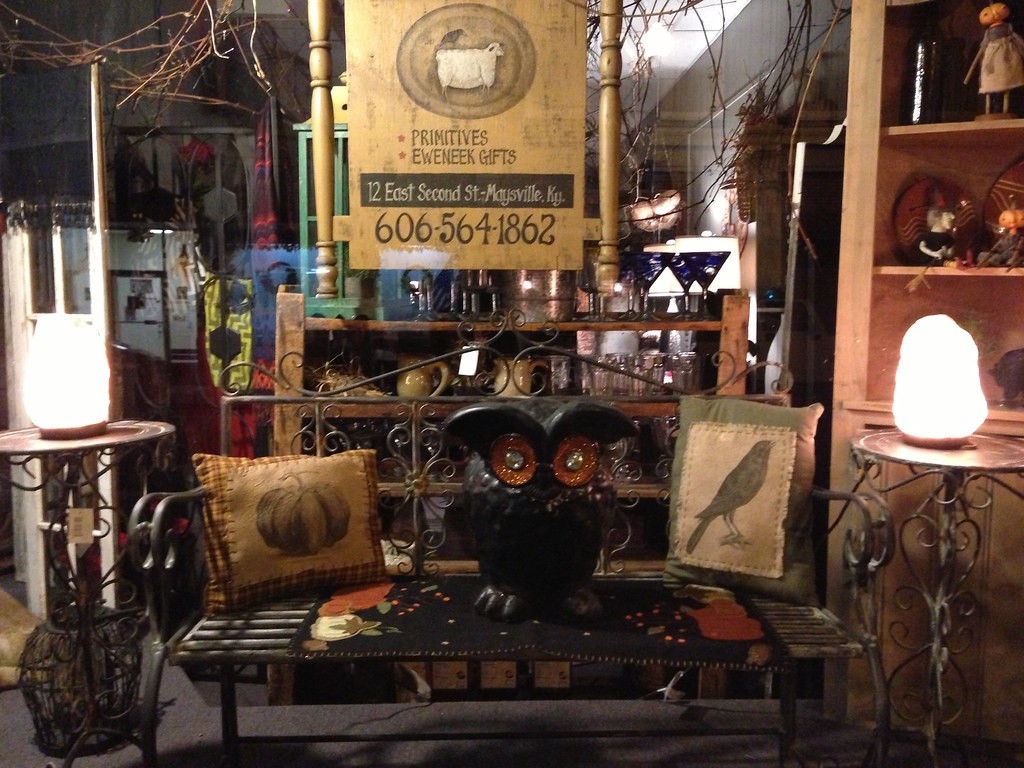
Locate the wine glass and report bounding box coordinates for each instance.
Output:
[624,252,675,322]
[681,251,731,321]
[668,256,698,321]
[615,255,641,322]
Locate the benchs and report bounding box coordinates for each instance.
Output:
[167,602,862,766]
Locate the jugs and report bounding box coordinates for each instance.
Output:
[491,359,547,397]
[394,356,448,396]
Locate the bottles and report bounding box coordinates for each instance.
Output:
[901,0,948,124]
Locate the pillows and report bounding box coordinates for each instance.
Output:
[659,391,831,599]
[189,447,387,615]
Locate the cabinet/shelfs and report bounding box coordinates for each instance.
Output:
[271,287,750,575]
[819,0,1024,744]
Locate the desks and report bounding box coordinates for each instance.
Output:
[1,420,177,766]
[855,428,1024,765]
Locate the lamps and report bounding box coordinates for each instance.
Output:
[889,314,988,450]
[645,242,686,294]
[25,310,112,441]
[674,230,743,291]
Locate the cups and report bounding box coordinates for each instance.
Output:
[651,354,668,392]
[671,356,682,388]
[636,355,654,396]
[680,353,700,393]
[544,354,570,393]
[579,354,608,397]
[607,352,634,396]
